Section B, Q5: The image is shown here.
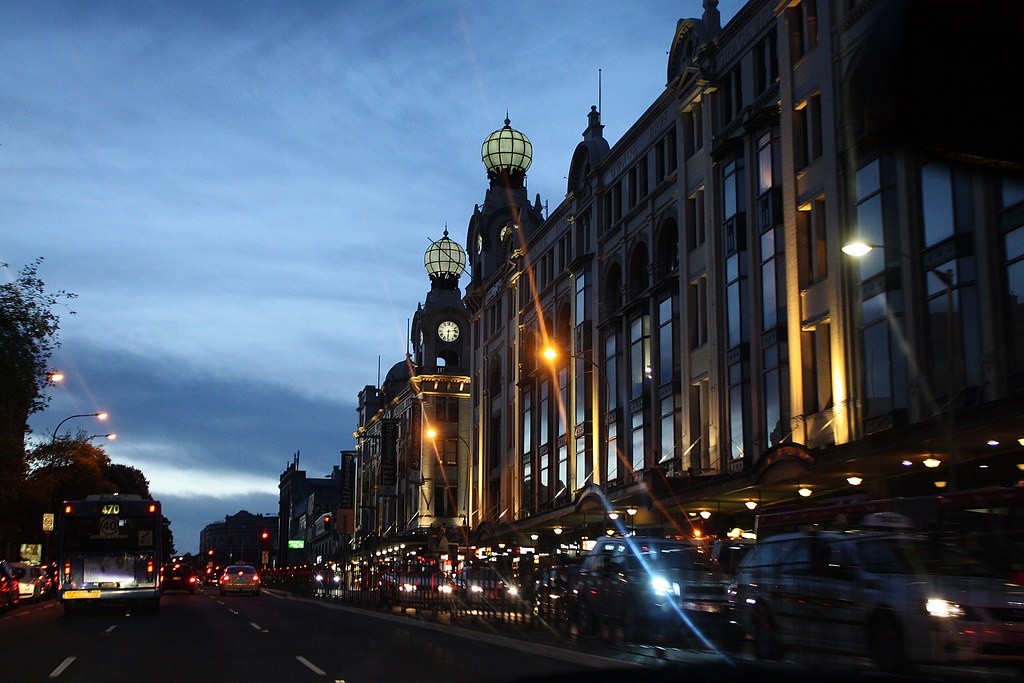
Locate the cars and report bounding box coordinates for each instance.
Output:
[205,568,220,588]
[218,563,262,596]
[0,559,20,612]
[724,515,1024,668]
[163,563,195,593]
[8,560,57,600]
[315,566,342,593]
[441,562,519,609]
[534,564,582,622]
[379,559,457,613]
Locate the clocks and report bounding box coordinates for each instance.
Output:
[438,321,460,343]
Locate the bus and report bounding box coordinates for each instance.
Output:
[58,493,164,607]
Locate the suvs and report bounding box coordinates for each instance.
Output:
[575,532,739,646]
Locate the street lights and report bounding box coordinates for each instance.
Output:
[841,234,956,526]
[424,426,471,532]
[49,410,118,460]
[541,345,611,521]
